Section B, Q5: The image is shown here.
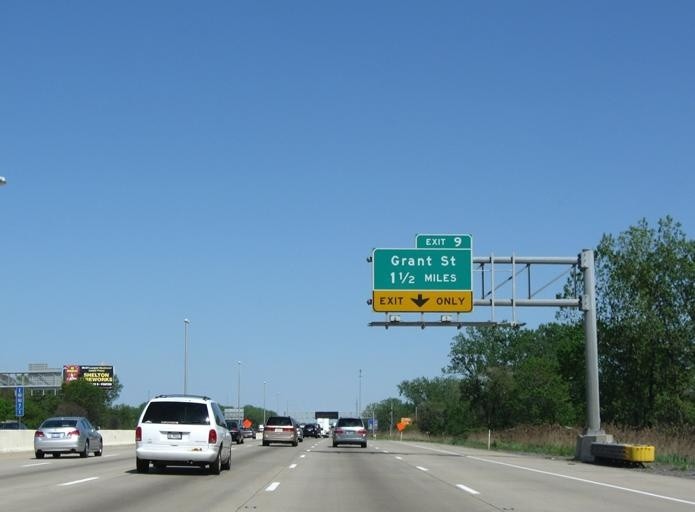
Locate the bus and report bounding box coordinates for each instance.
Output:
[315,412,339,438]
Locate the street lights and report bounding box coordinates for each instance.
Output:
[263,381,267,424]
[238,361,241,420]
[182,317,191,395]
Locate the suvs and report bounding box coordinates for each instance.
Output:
[134,395,233,477]
[226,419,244,444]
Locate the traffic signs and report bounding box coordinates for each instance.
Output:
[415,233,472,250]
[372,248,473,313]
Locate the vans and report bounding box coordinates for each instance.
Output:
[258,425,264,432]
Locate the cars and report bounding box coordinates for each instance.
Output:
[32,416,105,458]
[0,420,29,430]
[243,427,256,439]
[263,415,298,448]
[331,417,368,449]
[295,423,322,442]
